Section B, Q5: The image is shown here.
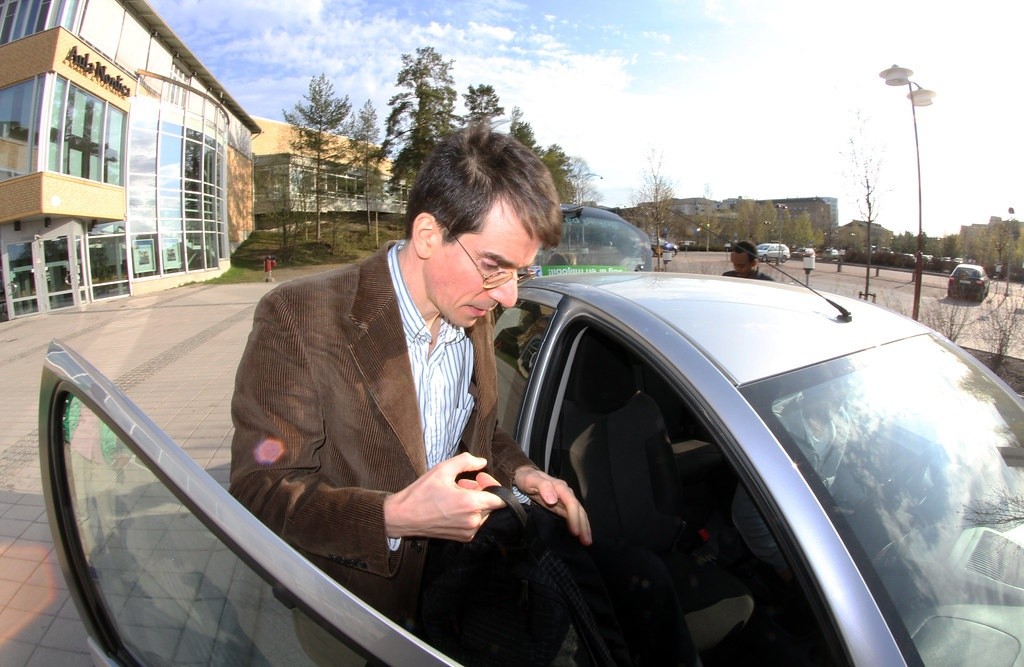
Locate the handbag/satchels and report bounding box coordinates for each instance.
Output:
[416,481,635,667]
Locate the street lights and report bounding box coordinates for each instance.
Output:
[581,174,603,242]
[880,64,937,320]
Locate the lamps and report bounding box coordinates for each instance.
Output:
[14,221,21,231]
[45,217,51,227]
[92,220,97,228]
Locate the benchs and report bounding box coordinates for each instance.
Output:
[632,360,726,488]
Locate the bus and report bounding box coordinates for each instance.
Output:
[488,203,654,279]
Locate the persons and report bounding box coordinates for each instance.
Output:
[225,119,595,667]
[721,240,775,281]
[731,372,897,569]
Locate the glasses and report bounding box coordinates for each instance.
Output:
[438,217,538,289]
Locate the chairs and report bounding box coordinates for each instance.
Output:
[552,342,757,648]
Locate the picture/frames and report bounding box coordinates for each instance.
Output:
[162,238,181,269]
[132,239,156,273]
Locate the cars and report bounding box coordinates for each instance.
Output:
[679,239,976,268]
[947,263,991,303]
[37,274,1024,667]
[754,243,791,263]
[649,236,680,258]
